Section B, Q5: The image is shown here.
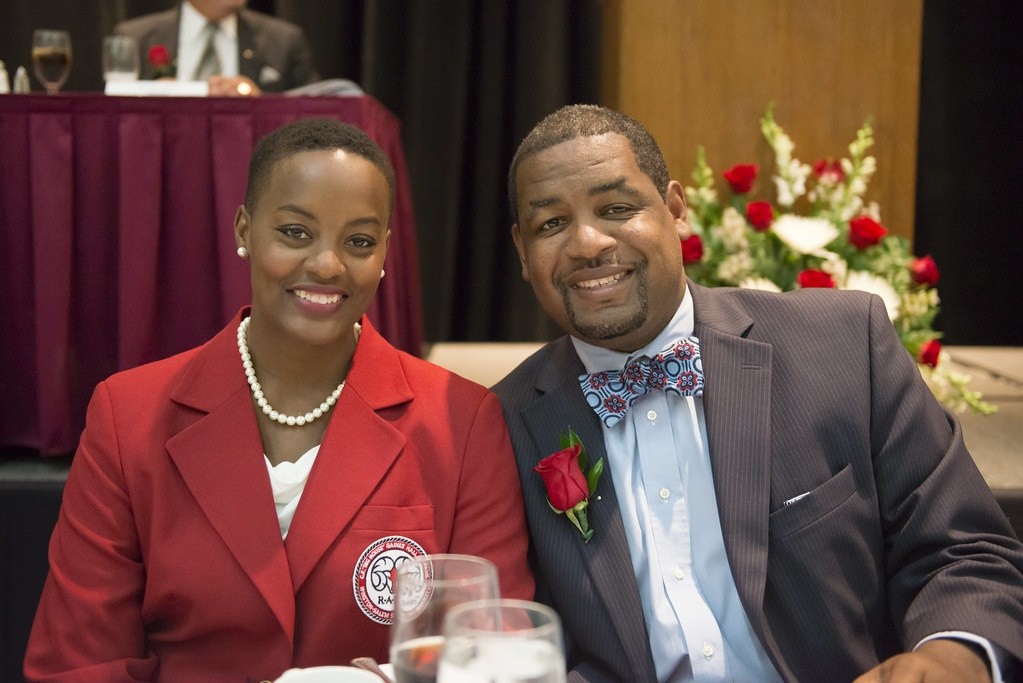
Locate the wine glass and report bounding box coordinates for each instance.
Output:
[31,29,74,95]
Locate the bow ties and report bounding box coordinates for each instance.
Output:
[577,337,706,430]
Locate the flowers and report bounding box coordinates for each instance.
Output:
[680,100,999,417]
[530,425,603,545]
[147,44,176,79]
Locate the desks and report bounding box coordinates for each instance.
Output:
[0,93,426,459]
[424,345,1022,506]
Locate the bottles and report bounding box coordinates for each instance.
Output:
[0,60,31,94]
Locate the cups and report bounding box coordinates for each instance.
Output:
[101,36,142,83]
[388,551,502,683]
[435,597,567,683]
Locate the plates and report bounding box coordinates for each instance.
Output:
[271,662,396,683]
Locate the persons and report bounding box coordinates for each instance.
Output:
[23,119,536,683]
[489,104,1023,683]
[115,0,319,96]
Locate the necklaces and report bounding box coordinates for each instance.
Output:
[237,315,362,426]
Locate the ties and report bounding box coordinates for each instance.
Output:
[190,17,224,78]
